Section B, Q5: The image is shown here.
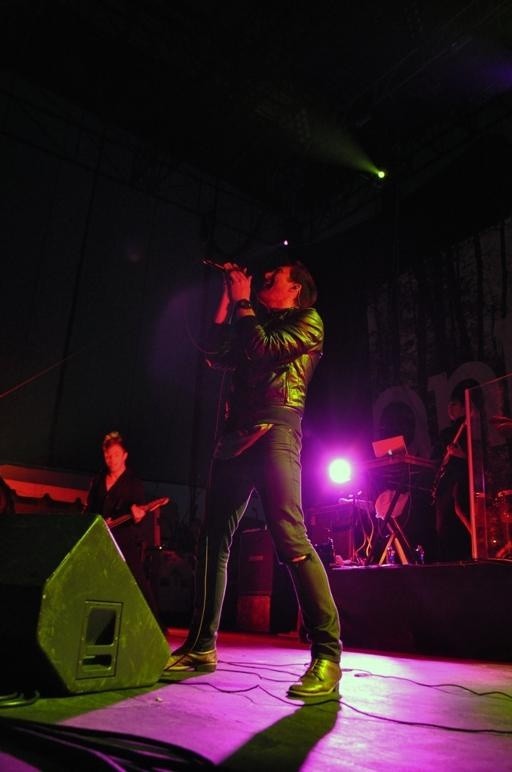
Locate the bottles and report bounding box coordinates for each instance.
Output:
[416,544,425,565]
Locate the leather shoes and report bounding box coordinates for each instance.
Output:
[164,647,218,673]
[287,659,342,696]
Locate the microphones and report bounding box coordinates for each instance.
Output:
[203,260,227,274]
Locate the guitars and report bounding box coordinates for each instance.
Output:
[106,497,169,529]
[429,409,473,508]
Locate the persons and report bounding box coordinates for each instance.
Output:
[81,431,147,587]
[430,380,488,560]
[160,261,344,696]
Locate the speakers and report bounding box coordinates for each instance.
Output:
[0,511,172,694]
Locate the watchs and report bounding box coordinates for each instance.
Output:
[232,298,251,310]
